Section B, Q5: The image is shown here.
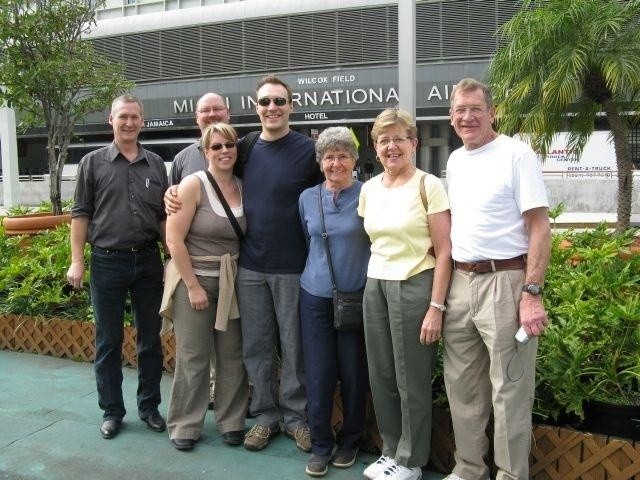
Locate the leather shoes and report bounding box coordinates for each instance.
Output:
[139,407,165,432]
[100,408,123,439]
[222,430,244,445]
[171,438,195,450]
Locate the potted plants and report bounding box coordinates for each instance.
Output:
[0,0,137,250]
[487,0,640,269]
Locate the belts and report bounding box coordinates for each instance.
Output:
[450,253,527,274]
[91,245,158,254]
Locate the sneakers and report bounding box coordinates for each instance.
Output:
[362,455,422,480]
[244,419,281,451]
[305,443,337,476]
[442,466,491,480]
[285,420,313,452]
[332,435,359,468]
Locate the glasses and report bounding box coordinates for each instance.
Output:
[257,97,290,107]
[374,136,413,146]
[205,142,235,151]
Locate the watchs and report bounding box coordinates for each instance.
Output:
[522,283,544,296]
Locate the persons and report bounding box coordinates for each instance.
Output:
[164,121,248,453]
[355,106,452,480]
[64,93,171,439]
[443,77,552,480]
[162,75,326,454]
[298,123,371,478]
[167,92,260,419]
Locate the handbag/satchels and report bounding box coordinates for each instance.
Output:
[333,289,365,332]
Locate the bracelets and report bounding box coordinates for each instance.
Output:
[429,301,447,313]
